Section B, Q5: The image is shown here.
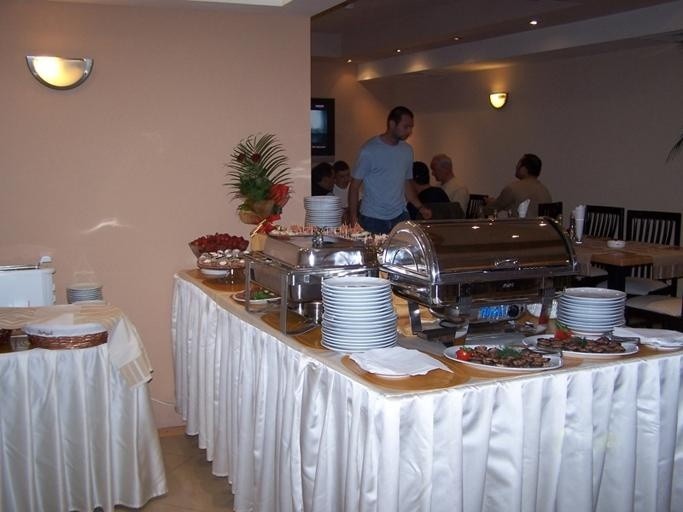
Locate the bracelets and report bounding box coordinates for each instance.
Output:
[418,204,424,210]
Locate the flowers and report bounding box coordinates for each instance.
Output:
[222,128,296,224]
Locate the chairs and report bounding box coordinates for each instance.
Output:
[463,194,683,332]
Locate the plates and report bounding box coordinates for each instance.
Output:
[319,275,400,354]
[355,347,429,379]
[65,284,104,303]
[526,301,558,320]
[556,286,626,336]
[441,333,639,371]
[231,289,280,305]
[302,195,343,228]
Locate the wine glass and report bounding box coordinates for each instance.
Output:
[572,208,586,245]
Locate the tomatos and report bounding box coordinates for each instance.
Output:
[456,346,474,361]
[555,329,573,341]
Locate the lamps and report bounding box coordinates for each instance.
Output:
[489,93,508,109]
[25,54,94,90]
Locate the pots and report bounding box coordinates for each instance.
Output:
[0,262,55,307]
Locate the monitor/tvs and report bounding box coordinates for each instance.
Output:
[310,97,335,160]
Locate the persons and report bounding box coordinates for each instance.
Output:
[332,160,364,209]
[348,105,433,234]
[482,153,552,218]
[406,161,451,219]
[430,154,470,217]
[311,162,336,196]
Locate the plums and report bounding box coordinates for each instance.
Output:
[191,233,249,252]
